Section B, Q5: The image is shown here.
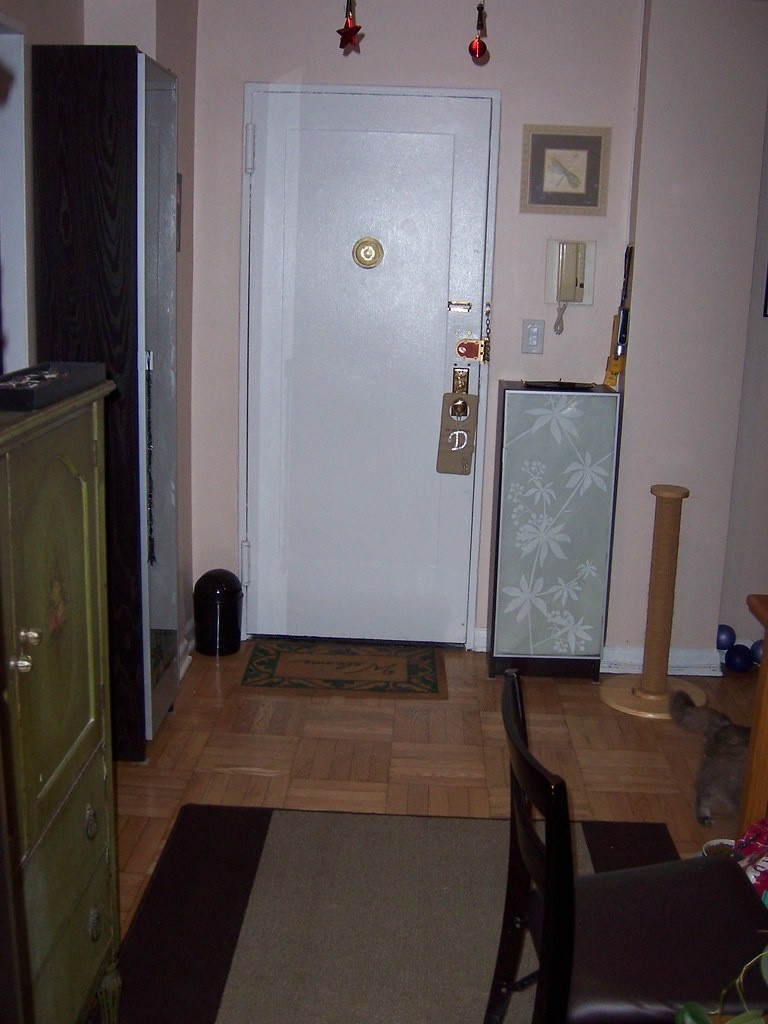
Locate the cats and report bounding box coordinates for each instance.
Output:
[671,691,768,826]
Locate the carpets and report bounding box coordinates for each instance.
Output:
[114,802,680,1024]
[239,634,449,700]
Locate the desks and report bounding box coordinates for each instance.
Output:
[737,595,768,838]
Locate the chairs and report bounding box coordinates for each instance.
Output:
[482,670,768,1024]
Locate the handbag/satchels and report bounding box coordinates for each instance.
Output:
[732,818,768,910]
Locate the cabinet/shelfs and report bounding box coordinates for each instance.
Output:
[30,40,193,762]
[485,379,620,683]
[0,376,117,1024]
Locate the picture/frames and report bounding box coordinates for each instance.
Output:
[518,123,612,218]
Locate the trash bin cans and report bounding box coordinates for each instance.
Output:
[190,568,243,658]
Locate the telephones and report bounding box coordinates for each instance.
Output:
[545,237,596,307]
